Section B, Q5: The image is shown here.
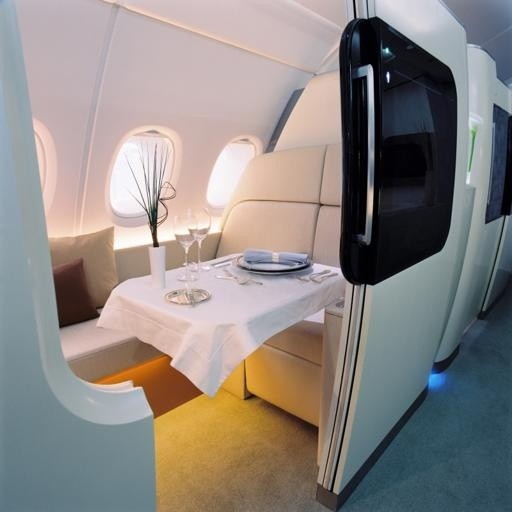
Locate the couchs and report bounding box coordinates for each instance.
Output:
[60,144,342,427]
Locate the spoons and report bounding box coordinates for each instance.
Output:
[309,273,338,282]
[225,268,250,284]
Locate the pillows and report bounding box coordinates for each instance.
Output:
[52,257,100,328]
[48,226,119,309]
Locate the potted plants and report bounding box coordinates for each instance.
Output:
[118,138,177,290]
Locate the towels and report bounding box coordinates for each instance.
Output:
[244,248,308,264]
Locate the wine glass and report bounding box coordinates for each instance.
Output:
[187,208,211,272]
[174,215,199,281]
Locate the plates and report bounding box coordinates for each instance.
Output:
[165,288,211,305]
[236,253,312,275]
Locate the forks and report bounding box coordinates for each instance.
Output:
[295,269,331,282]
